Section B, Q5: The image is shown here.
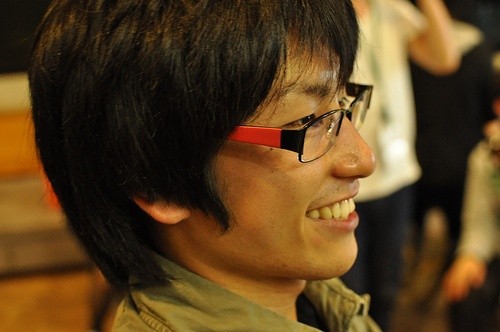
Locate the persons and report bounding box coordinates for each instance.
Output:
[438,75,499,332]
[27,0,383,332]
[341,0,460,332]
[393,1,500,323]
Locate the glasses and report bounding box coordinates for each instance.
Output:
[226,82,373,163]
